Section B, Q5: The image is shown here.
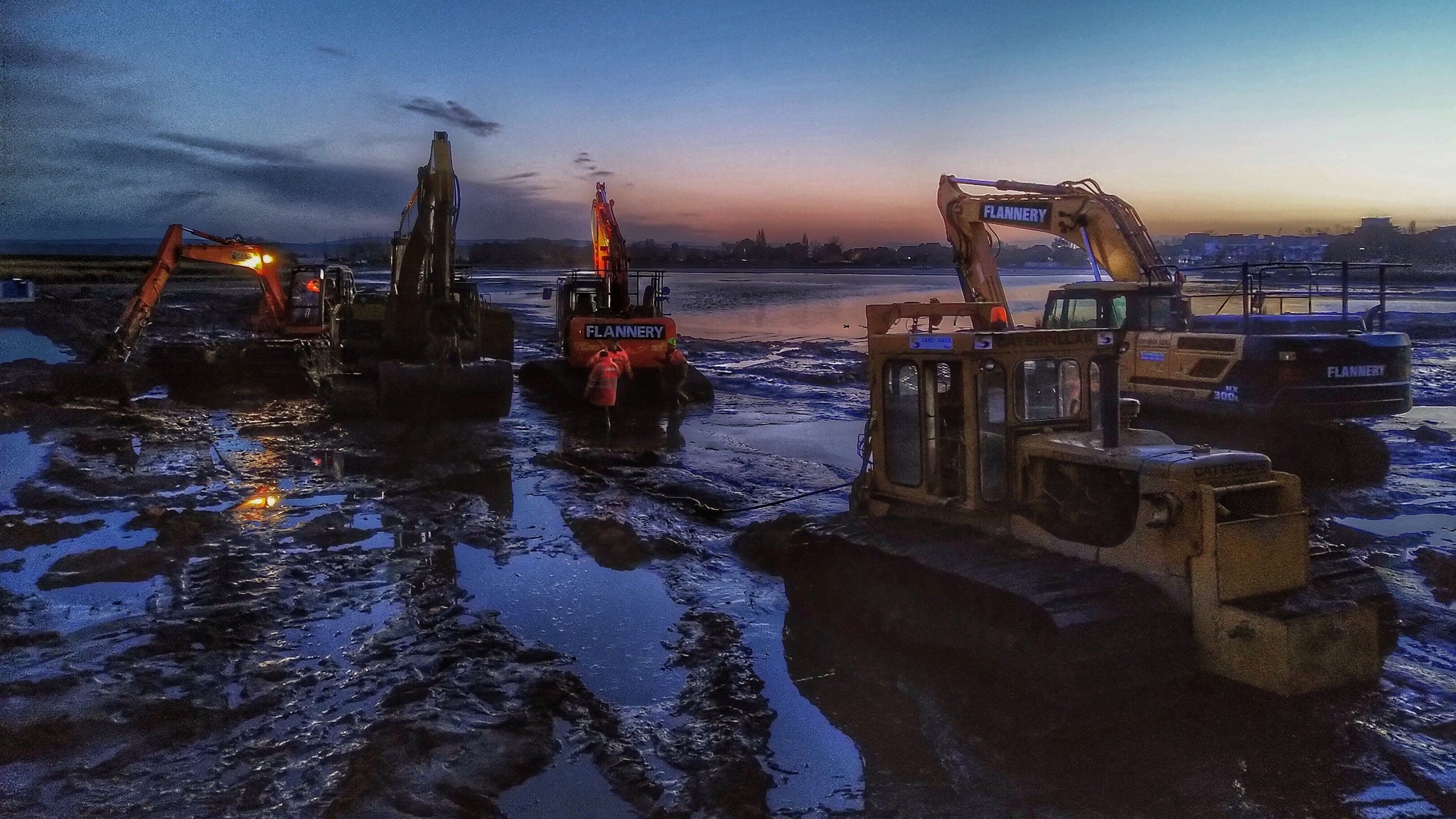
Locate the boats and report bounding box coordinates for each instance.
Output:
[0,279,38,313]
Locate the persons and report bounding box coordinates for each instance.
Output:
[617,296,645,319]
[585,336,634,413]
[322,278,337,311]
[655,337,691,411]
[583,350,621,440]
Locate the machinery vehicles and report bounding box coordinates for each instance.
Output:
[779,299,1403,712]
[517,182,717,418]
[82,222,359,397]
[936,172,1414,485]
[318,131,517,418]
